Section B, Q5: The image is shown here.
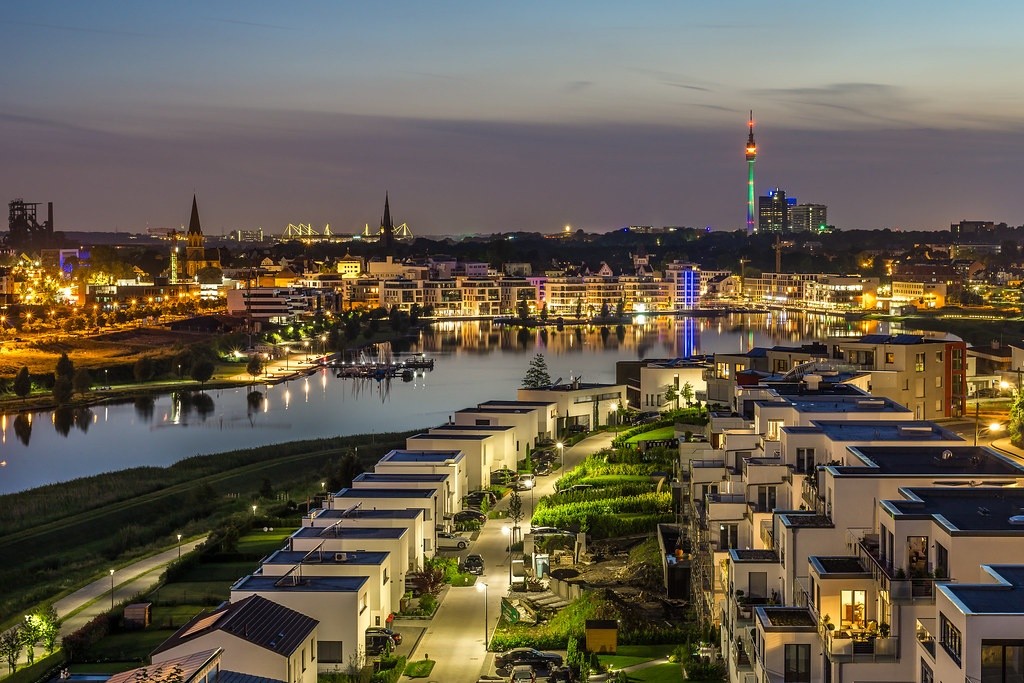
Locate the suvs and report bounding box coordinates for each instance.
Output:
[438,531,470,549]
[366,627,403,646]
[466,490,497,508]
[516,473,536,491]
[365,633,396,653]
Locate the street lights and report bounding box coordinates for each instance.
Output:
[974,402,1001,445]
[477,581,489,651]
[252,505,257,516]
[177,534,182,560]
[502,525,513,585]
[110,569,117,610]
[521,479,535,519]
[556,443,565,477]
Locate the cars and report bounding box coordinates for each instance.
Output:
[975,388,1003,398]
[533,460,553,477]
[530,526,570,538]
[490,469,517,484]
[406,570,437,595]
[495,647,564,675]
[524,438,560,464]
[454,510,487,524]
[464,554,486,575]
